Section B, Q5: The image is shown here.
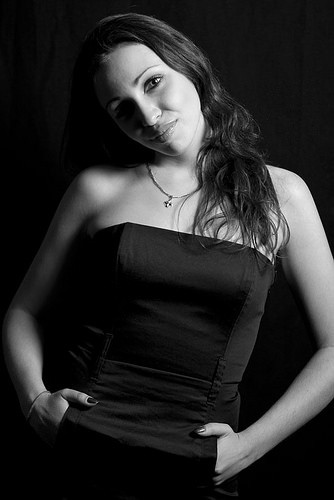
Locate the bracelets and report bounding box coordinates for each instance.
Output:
[22,389,52,424]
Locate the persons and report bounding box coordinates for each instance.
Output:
[1,9,334,500]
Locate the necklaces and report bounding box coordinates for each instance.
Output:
[142,158,204,209]
